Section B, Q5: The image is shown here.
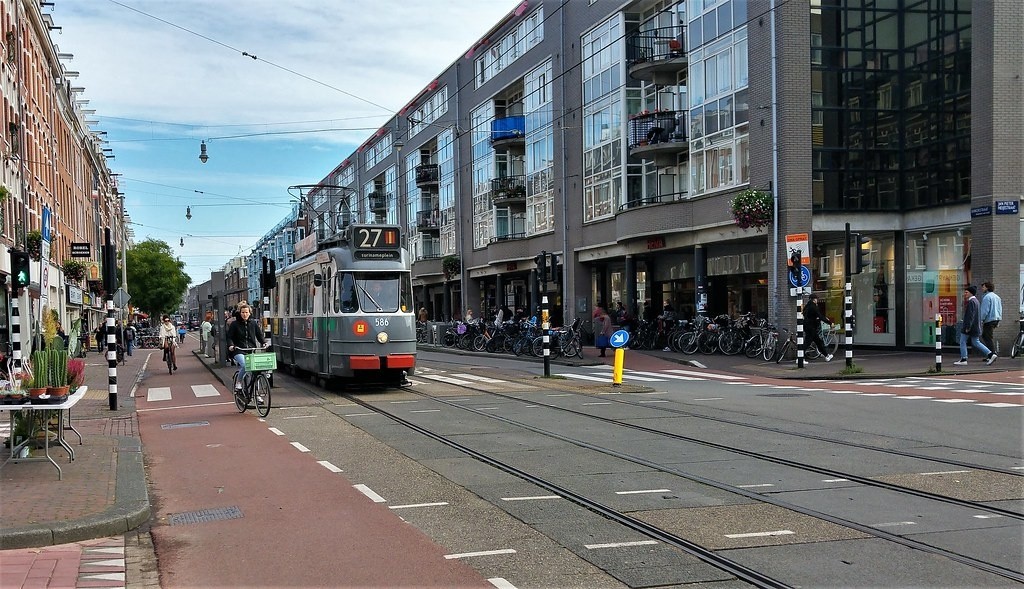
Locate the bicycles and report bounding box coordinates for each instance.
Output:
[1010,320,1024,358]
[180,331,186,344]
[625,316,839,365]
[415,317,596,359]
[162,337,175,375]
[231,343,278,417]
[105,328,164,364]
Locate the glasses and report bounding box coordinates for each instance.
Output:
[165,320,170,322]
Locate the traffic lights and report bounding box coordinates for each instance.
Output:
[856,237,871,268]
[534,255,546,281]
[792,252,801,275]
[11,252,30,288]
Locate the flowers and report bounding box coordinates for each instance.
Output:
[726,189,774,234]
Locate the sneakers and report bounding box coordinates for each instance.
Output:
[987,353,997,365]
[953,358,967,365]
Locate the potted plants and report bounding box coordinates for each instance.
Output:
[27,346,70,398]
[0,387,26,398]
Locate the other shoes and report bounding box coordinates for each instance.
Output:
[236,380,243,390]
[796,358,809,364]
[982,358,990,362]
[663,347,671,352]
[256,395,264,405]
[825,354,834,362]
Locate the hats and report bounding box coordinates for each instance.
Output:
[809,294,817,299]
[965,286,977,296]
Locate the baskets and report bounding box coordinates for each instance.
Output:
[244,353,277,372]
[179,329,186,334]
[457,325,466,334]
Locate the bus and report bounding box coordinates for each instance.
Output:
[269,220,417,391]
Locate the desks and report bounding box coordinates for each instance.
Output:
[0,385,88,481]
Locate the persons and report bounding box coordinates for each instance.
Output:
[802,293,836,364]
[200,316,213,357]
[466,309,476,325]
[158,315,179,371]
[494,306,510,326]
[980,281,1003,362]
[93,315,149,356]
[593,295,691,357]
[180,325,186,342]
[205,300,247,367]
[416,306,428,325]
[953,286,998,366]
[226,300,269,404]
[371,282,388,310]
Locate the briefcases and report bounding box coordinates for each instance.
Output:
[595,334,610,348]
